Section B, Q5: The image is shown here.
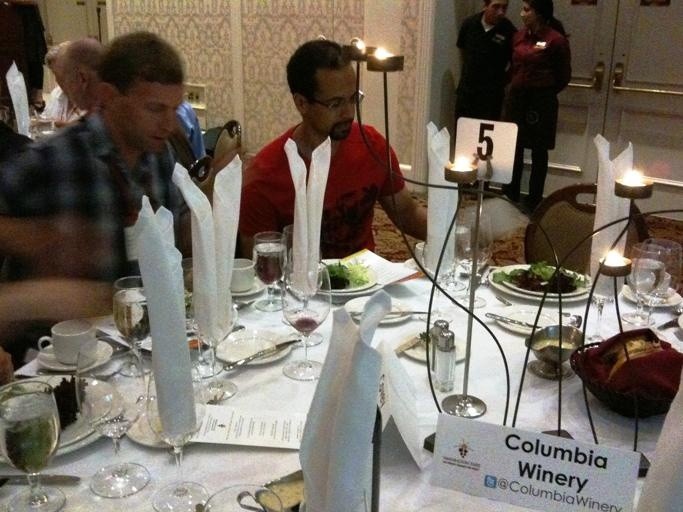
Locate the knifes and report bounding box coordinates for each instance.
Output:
[223,339,300,372]
[0,474,82,488]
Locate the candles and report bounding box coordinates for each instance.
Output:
[602,250,628,266]
[451,156,474,172]
[372,47,394,60]
[618,170,646,186]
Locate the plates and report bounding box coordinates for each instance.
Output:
[344,297,411,326]
[496,305,571,337]
[37,340,114,372]
[400,331,467,363]
[623,285,682,308]
[1,373,123,464]
[315,266,382,295]
[225,281,266,297]
[215,328,292,367]
[488,264,593,303]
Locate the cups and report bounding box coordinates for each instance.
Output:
[204,466,308,512]
[414,240,428,269]
[231,256,256,292]
[37,318,95,363]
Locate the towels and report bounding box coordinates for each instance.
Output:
[170,154,242,344]
[425,122,457,279]
[589,132,633,296]
[284,136,331,299]
[297,287,394,512]
[6,62,31,137]
[135,194,199,434]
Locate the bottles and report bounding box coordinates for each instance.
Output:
[428,320,456,393]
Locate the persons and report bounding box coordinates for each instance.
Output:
[38,37,206,160]
[0,31,191,386]
[239,41,427,260]
[0,0,48,130]
[454,0,572,209]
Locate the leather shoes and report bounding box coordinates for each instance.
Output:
[501,182,521,202]
[521,192,543,211]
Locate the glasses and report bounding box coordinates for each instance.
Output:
[308,91,365,112]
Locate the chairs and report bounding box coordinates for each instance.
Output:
[179,155,212,209]
[209,120,241,187]
[527,182,650,285]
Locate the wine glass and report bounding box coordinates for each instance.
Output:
[586,236,682,349]
[525,323,583,382]
[77,259,235,512]
[420,209,492,326]
[1,380,67,510]
[254,224,331,383]
